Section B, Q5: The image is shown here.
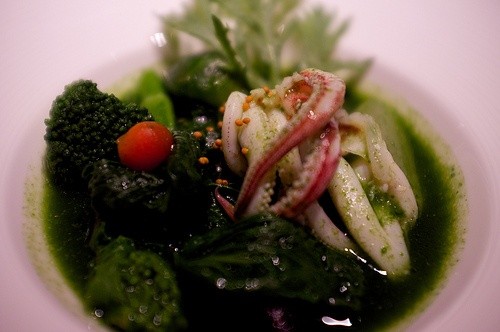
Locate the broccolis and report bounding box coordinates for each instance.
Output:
[40,80,156,194]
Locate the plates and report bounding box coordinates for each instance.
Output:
[0,1,499,332]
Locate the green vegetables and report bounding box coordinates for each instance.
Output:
[159,0,375,102]
